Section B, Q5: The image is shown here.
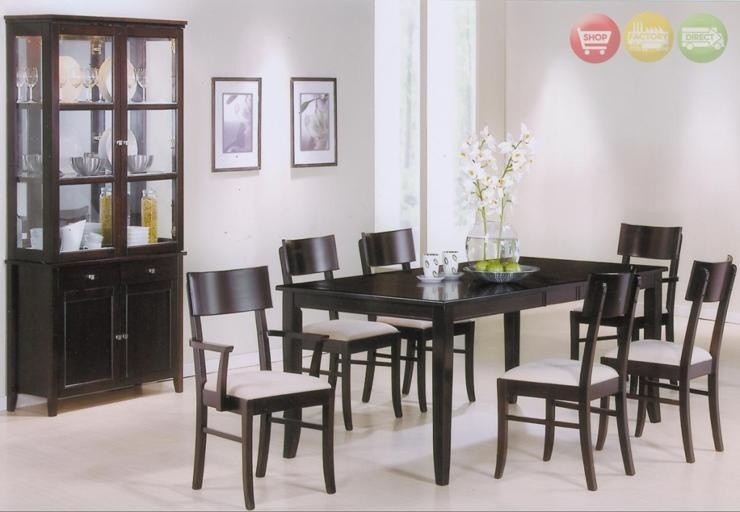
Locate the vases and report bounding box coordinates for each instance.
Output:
[464,218,522,268]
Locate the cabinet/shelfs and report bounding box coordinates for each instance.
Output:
[2,10,189,266]
[2,250,189,418]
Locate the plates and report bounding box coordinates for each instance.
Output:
[464,265,541,284]
[104,58,137,103]
[99,59,113,103]
[105,127,140,170]
[96,127,111,162]
[60,55,81,101]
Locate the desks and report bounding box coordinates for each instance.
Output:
[276,255,672,490]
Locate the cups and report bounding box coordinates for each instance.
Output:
[416,251,463,283]
[421,282,459,301]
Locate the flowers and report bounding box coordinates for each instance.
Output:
[452,120,533,262]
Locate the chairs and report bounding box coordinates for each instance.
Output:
[592,252,740,466]
[183,265,340,510]
[276,233,403,430]
[356,226,478,414]
[567,222,685,390]
[489,264,645,494]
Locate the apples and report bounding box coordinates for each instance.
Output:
[476,259,521,273]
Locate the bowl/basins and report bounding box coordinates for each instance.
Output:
[27,219,102,251]
[22,154,153,177]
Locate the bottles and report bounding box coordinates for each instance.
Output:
[98,188,159,247]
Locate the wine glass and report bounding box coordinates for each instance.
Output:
[16,66,151,103]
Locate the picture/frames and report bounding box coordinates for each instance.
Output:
[208,75,264,175]
[287,75,341,172]
